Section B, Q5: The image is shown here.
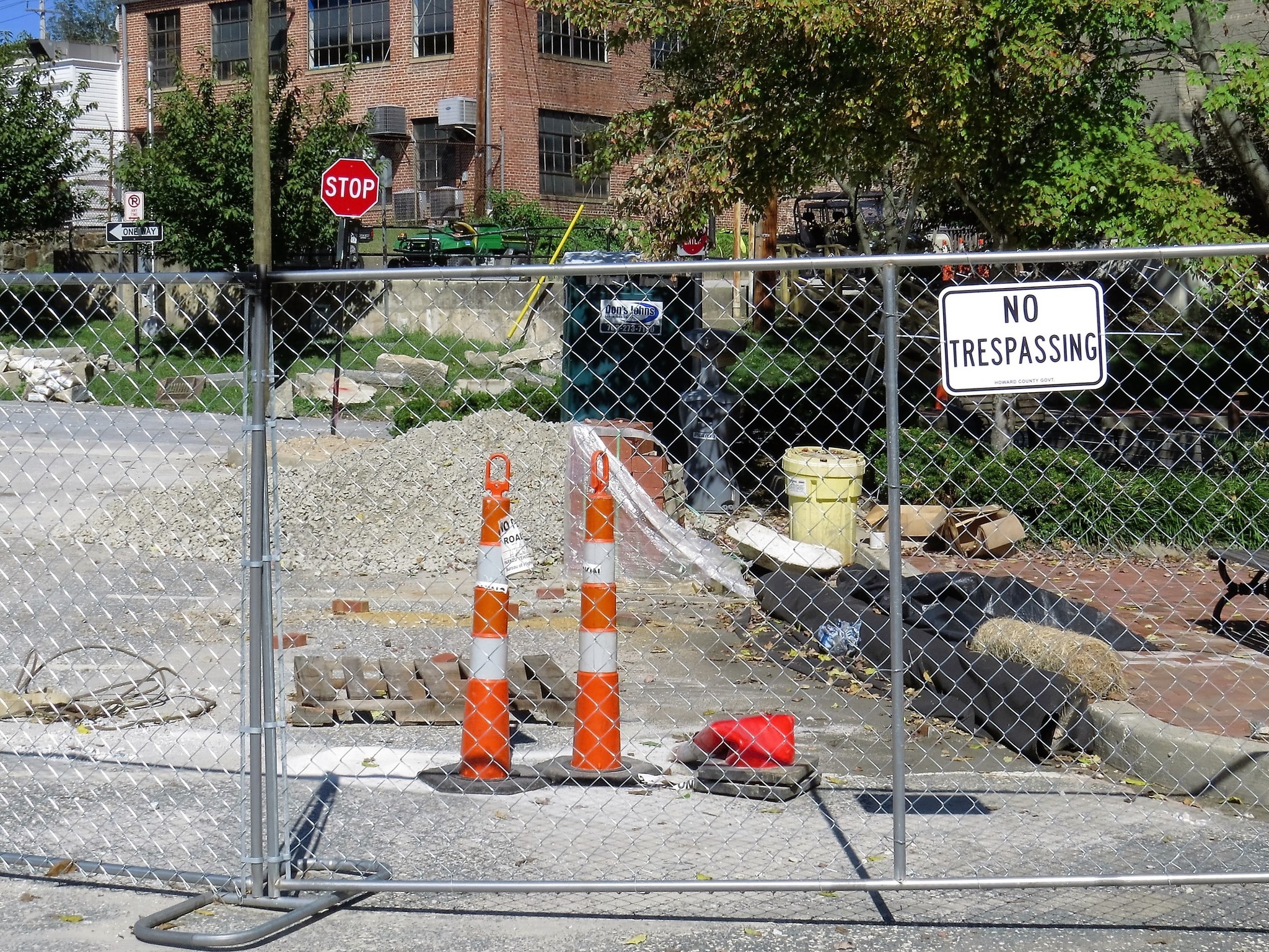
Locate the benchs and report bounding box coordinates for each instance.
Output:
[1207,549,1269,635]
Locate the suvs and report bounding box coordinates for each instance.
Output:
[387,221,532,269]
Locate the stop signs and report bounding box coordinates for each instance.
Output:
[677,219,709,256]
[321,158,379,217]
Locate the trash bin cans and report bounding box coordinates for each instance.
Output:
[561,249,696,464]
[782,445,866,567]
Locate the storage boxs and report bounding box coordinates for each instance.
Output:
[866,503,1026,559]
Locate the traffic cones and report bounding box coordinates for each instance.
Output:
[976,237,989,278]
[941,240,955,282]
[932,359,949,413]
[957,237,973,276]
[670,713,820,780]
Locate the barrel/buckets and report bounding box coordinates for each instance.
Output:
[782,446,866,567]
[870,531,886,549]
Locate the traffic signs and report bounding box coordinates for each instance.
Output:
[105,220,164,243]
